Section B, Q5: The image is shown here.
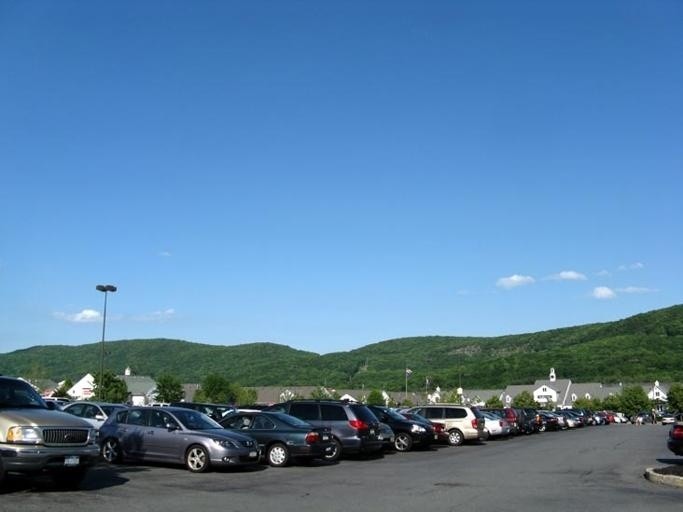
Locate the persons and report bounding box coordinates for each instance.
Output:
[629,406,658,431]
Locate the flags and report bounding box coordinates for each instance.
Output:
[426,377,433,386]
[406,368,413,377]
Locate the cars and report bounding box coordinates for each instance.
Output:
[661,413,683,456]
[630,411,654,424]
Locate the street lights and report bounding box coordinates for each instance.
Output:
[405,367,413,398]
[95,284,117,401]
